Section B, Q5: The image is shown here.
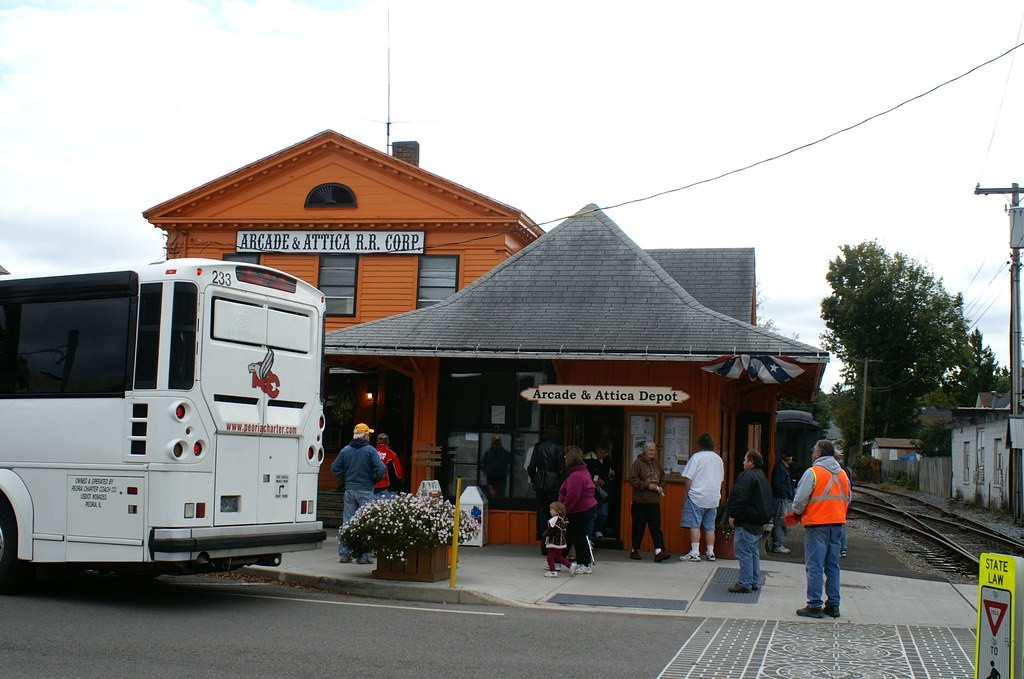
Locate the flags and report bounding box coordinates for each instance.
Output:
[697,354,809,384]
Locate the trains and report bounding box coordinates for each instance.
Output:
[776,410,825,488]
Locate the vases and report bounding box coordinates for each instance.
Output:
[371,545,451,582]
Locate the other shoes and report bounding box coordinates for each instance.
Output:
[595,531,602,536]
[340,554,352,563]
[728,582,759,592]
[840,551,846,556]
[358,556,375,564]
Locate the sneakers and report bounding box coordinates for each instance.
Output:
[824,604,840,617]
[680,550,701,561]
[543,562,561,570]
[569,563,577,576]
[574,565,591,575]
[703,551,716,561]
[545,571,557,577]
[796,604,824,618]
[655,550,671,562]
[631,548,641,559]
[773,545,791,553]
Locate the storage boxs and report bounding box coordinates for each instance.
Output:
[459,486,488,547]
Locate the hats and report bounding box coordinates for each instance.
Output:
[833,449,844,456]
[378,433,389,443]
[490,434,500,442]
[354,423,376,436]
[783,449,795,458]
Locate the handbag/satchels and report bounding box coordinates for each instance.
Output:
[594,484,610,507]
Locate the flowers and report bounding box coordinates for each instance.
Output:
[338,490,478,561]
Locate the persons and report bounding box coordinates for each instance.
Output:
[791,439,851,618]
[527,422,618,578]
[481,434,515,503]
[833,451,853,557]
[371,433,404,501]
[629,441,671,562]
[331,423,386,566]
[679,433,725,562]
[727,449,774,593]
[765,452,797,555]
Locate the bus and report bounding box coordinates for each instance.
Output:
[0,256,328,590]
